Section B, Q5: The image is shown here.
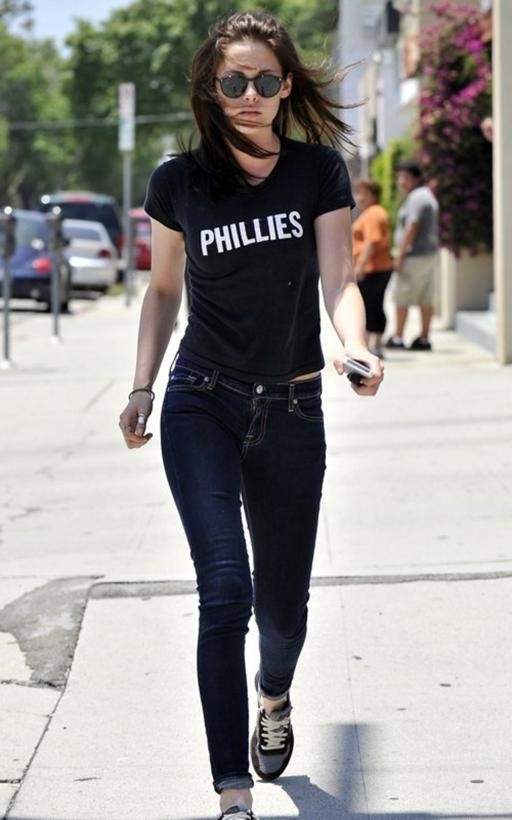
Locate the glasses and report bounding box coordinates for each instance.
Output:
[215,74,286,99]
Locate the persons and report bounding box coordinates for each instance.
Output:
[116,9,390,819]
[348,177,394,364]
[380,159,445,354]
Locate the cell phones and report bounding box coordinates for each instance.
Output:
[343,357,374,378]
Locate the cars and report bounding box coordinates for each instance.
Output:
[56,217,117,293]
[0,205,72,315]
[123,206,153,271]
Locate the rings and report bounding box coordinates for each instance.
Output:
[136,412,146,426]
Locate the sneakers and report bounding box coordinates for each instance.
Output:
[218,803,263,820]
[385,338,404,348]
[251,670,295,781]
[410,338,431,350]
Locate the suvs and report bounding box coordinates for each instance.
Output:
[37,190,126,285]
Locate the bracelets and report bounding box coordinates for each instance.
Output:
[126,388,156,402]
